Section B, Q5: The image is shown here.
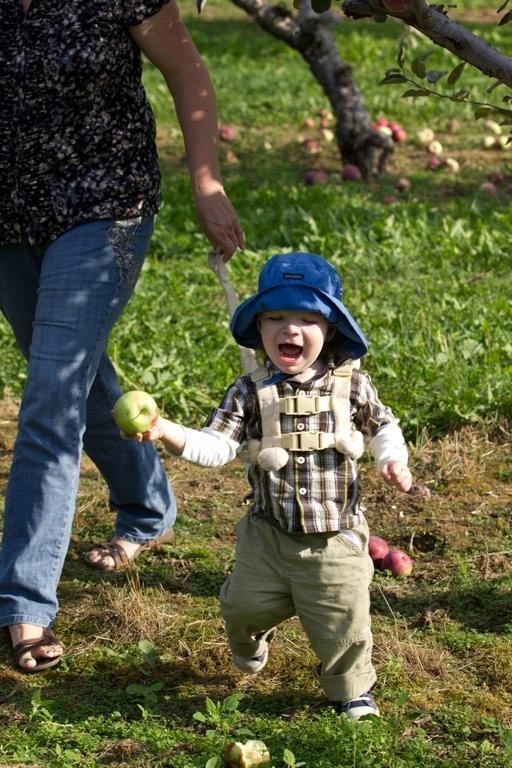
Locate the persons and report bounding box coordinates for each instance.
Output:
[110,250,417,722]
[0,0,246,672]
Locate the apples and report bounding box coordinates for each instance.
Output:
[382,550,413,577]
[218,109,512,205]
[223,739,271,768]
[113,390,161,437]
[368,535,389,568]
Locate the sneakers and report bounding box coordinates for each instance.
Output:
[312,661,380,722]
[229,625,279,672]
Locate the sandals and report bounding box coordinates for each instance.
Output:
[78,525,176,572]
[5,624,63,674]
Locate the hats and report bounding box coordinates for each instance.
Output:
[230,250,370,364]
